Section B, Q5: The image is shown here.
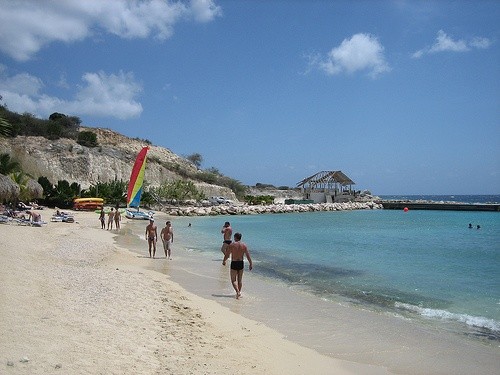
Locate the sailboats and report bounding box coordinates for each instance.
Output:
[125,145,153,220]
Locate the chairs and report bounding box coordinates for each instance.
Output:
[0,209,75,227]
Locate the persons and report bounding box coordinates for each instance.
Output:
[220,221,232,257]
[188,223,191,226]
[114,207,121,228]
[221,231,253,299]
[26,210,41,224]
[106,207,115,230]
[468,223,480,229]
[160,220,173,260]
[6,201,43,216]
[57,210,70,218]
[99,206,105,229]
[145,219,158,259]
